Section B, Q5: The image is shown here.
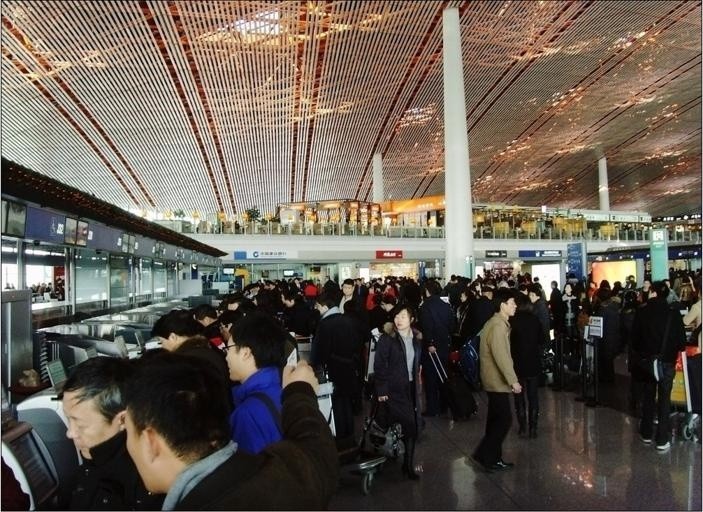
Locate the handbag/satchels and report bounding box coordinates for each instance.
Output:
[628,354,664,386]
[358,417,405,458]
[461,333,479,383]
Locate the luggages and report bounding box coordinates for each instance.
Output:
[429,352,479,420]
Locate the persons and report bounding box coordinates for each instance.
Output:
[1,262,702,510]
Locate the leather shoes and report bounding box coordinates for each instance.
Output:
[472,454,513,473]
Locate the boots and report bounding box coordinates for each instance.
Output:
[529,409,539,438]
[515,406,527,436]
[401,454,420,481]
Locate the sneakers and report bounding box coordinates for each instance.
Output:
[656,440,670,450]
[639,435,652,444]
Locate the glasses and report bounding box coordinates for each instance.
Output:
[221,344,236,353]
